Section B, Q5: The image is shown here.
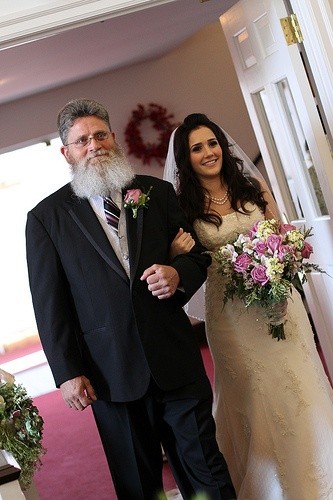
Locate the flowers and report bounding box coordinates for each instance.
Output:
[0,383,47,492]
[123,102,180,168]
[202,214,332,341]
[123,185,154,219]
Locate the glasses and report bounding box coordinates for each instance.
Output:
[65,130,111,148]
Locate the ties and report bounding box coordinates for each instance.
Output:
[98,189,121,237]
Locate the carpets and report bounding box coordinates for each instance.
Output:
[23,345,214,500]
